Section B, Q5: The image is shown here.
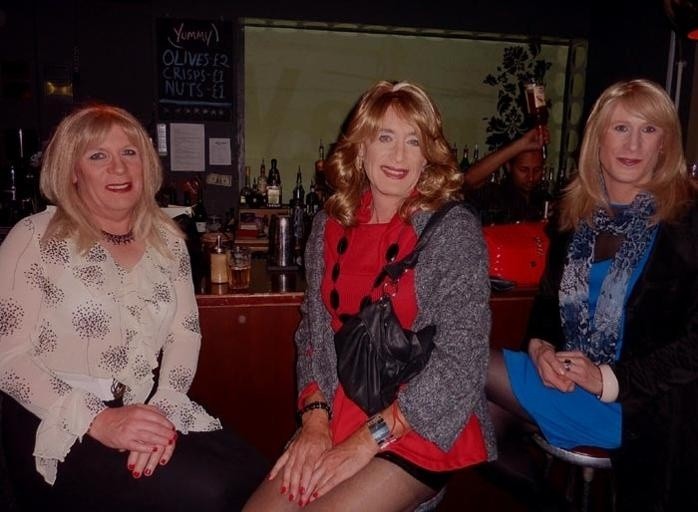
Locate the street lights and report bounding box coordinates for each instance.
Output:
[335,296,437,415]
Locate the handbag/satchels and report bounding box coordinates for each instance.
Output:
[595,364,603,402]
[298,400,331,426]
[366,413,397,452]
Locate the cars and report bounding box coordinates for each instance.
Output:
[227,247,251,289]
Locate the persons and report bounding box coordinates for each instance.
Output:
[240,82,498,512]
[0,104,270,511]
[462,127,555,223]
[485,79,698,511]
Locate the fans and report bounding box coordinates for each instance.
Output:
[155,16,233,123]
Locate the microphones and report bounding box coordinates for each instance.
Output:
[532,434,609,511]
[415,486,447,512]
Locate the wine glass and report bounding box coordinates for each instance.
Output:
[565,360,571,372]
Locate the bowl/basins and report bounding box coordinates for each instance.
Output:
[99,229,136,245]
[375,206,395,224]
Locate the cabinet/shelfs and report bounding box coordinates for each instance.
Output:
[450,143,497,184]
[240,139,327,269]
[523,76,549,160]
[169,188,208,233]
[210,235,230,283]
[540,166,568,220]
